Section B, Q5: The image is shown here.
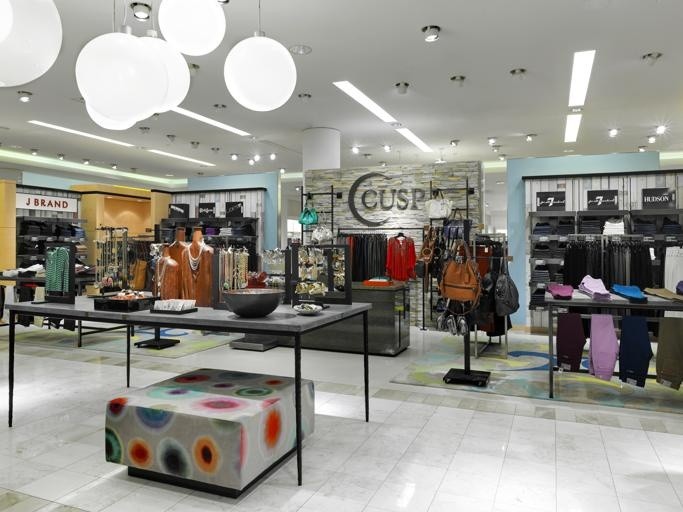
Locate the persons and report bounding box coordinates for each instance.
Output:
[166,226,187,300]
[155,247,178,301]
[181,227,214,307]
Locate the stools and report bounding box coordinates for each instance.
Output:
[104,368,316,499]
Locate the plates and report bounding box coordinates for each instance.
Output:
[294,305,321,315]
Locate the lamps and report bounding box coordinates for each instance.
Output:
[72,1,299,132]
[421,25,440,44]
[16,90,34,104]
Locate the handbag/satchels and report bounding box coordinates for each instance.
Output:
[414,226,446,278]
[440,242,479,301]
[443,208,472,239]
[425,189,453,219]
[311,227,333,243]
[299,208,318,225]
[479,272,519,317]
[295,282,325,295]
[436,303,497,335]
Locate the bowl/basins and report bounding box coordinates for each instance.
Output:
[220,287,285,316]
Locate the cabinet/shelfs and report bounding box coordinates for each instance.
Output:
[150,219,257,249]
[16,214,89,277]
[529,210,682,333]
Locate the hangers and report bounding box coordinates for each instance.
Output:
[386,228,412,243]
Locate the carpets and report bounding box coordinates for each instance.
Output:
[390,334,682,416]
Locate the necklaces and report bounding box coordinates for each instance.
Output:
[157,256,170,297]
[187,242,203,271]
[219,250,249,290]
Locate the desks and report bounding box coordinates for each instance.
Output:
[543,285,682,398]
[0,274,135,348]
[1,294,374,486]
